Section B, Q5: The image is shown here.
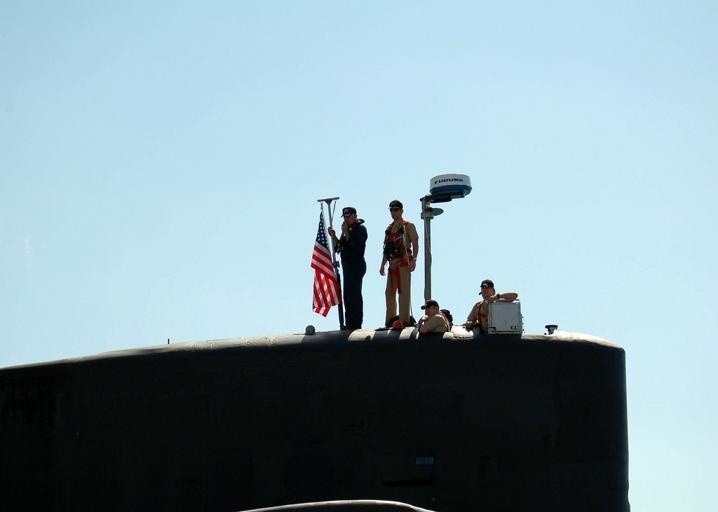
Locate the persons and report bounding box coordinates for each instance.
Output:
[466,280,518,328]
[380,200,418,327]
[419,300,450,334]
[328,208,368,331]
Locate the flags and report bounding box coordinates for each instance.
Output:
[310,204,340,317]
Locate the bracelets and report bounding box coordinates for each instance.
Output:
[413,255,416,261]
[496,294,500,299]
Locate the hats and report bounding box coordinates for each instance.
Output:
[481,280,494,288]
[341,207,356,217]
[421,299,439,309]
[389,199,403,210]
